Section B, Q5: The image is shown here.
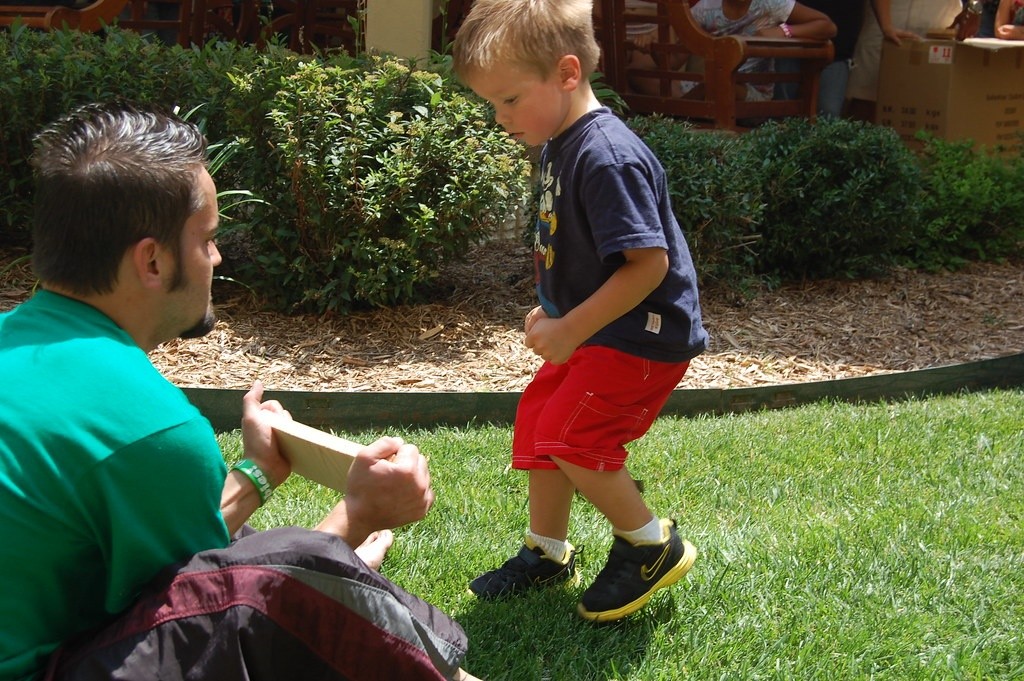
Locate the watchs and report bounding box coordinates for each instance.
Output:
[966,1,983,14]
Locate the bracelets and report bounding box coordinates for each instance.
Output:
[780,23,791,39]
[230,459,274,507]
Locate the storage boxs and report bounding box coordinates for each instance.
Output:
[874,37,1024,167]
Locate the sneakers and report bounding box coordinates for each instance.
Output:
[578,518,698,620]
[468,538,580,604]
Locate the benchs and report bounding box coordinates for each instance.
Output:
[594,0,834,132]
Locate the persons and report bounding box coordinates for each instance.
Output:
[625,0,838,102]
[452,0,707,619]
[993,0,1024,40]
[0,103,481,681]
[846,0,983,120]
